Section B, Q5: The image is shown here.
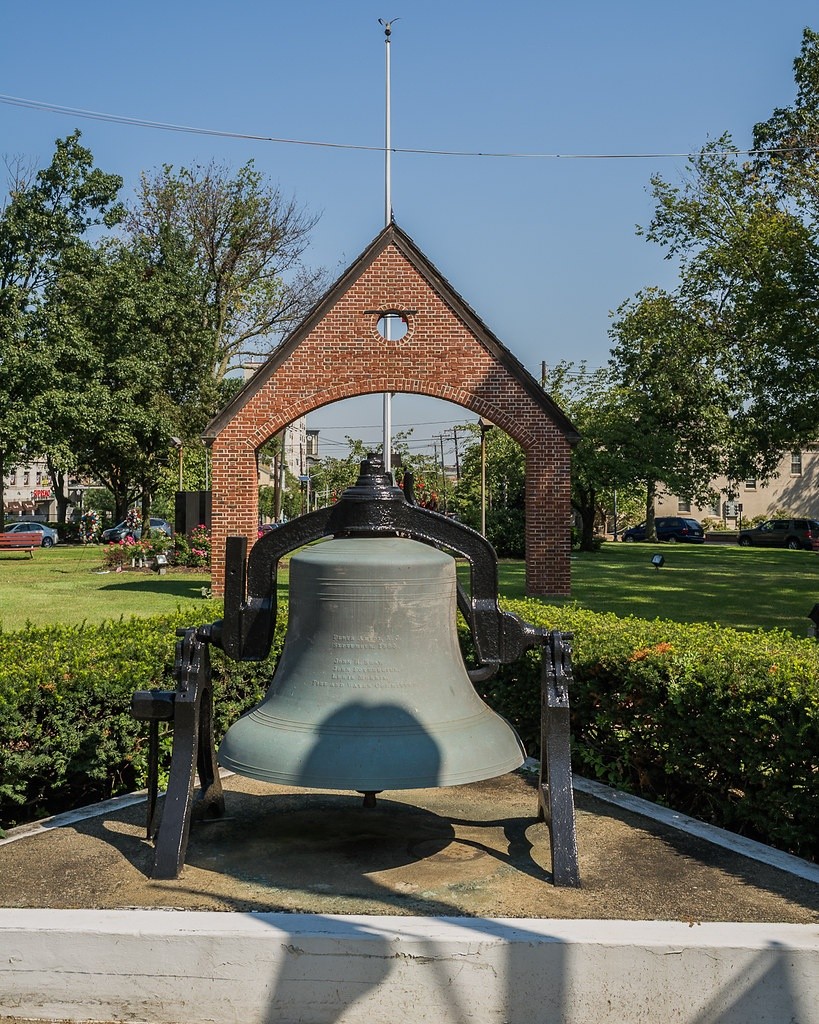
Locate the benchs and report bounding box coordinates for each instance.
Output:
[0,532,42,559]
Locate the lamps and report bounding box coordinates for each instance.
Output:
[808,602,819,629]
[154,555,169,568]
[651,554,665,568]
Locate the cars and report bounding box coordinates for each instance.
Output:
[100,517,172,546]
[4,522,58,548]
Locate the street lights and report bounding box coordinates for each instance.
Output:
[477,418,494,539]
[170,436,182,491]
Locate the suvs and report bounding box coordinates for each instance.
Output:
[736,518,819,550]
[622,516,705,543]
